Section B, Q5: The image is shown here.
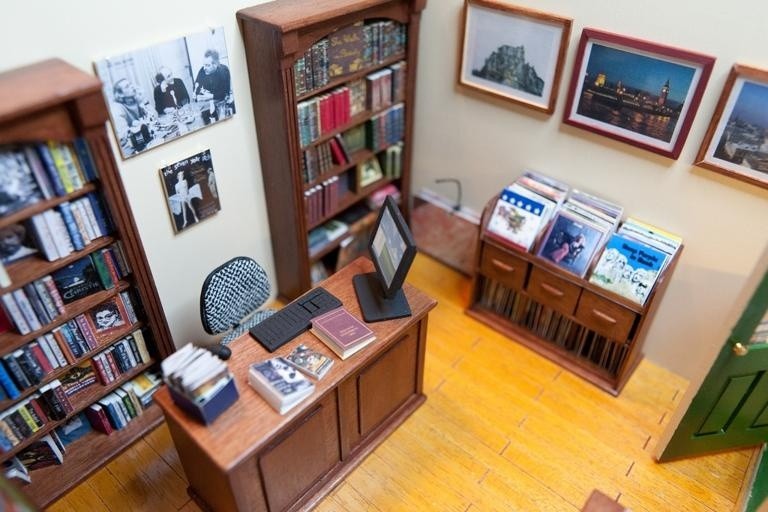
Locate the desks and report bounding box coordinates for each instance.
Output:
[151,250,443,512]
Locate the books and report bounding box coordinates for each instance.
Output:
[159,343,229,400]
[283,342,336,381]
[307,303,379,362]
[246,352,318,417]
[292,21,407,292]
[484,168,685,310]
[0,137,162,483]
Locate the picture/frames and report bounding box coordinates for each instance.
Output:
[694,61,767,194]
[450,0,574,119]
[560,27,719,162]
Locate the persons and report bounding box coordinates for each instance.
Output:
[152,66,190,117]
[192,48,230,103]
[167,171,203,231]
[206,168,220,210]
[111,78,149,150]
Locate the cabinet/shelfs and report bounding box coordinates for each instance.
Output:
[460,194,687,398]
[0,56,179,512]
[233,0,430,307]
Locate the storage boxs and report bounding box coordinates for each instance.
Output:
[165,371,242,427]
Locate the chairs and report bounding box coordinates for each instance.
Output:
[200,255,277,353]
